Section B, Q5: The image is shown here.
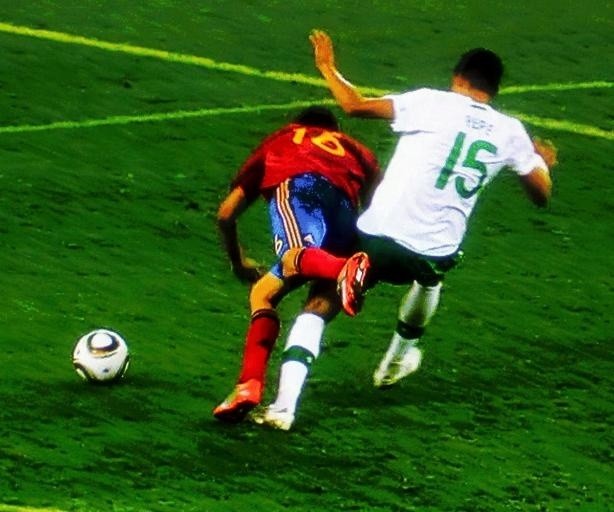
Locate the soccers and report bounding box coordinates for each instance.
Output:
[72,328,131,383]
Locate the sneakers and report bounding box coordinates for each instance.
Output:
[211,377,263,425]
[244,404,296,432]
[334,251,373,318]
[370,345,423,390]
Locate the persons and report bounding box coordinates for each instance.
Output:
[212,105,382,423]
[253,29,559,430]
